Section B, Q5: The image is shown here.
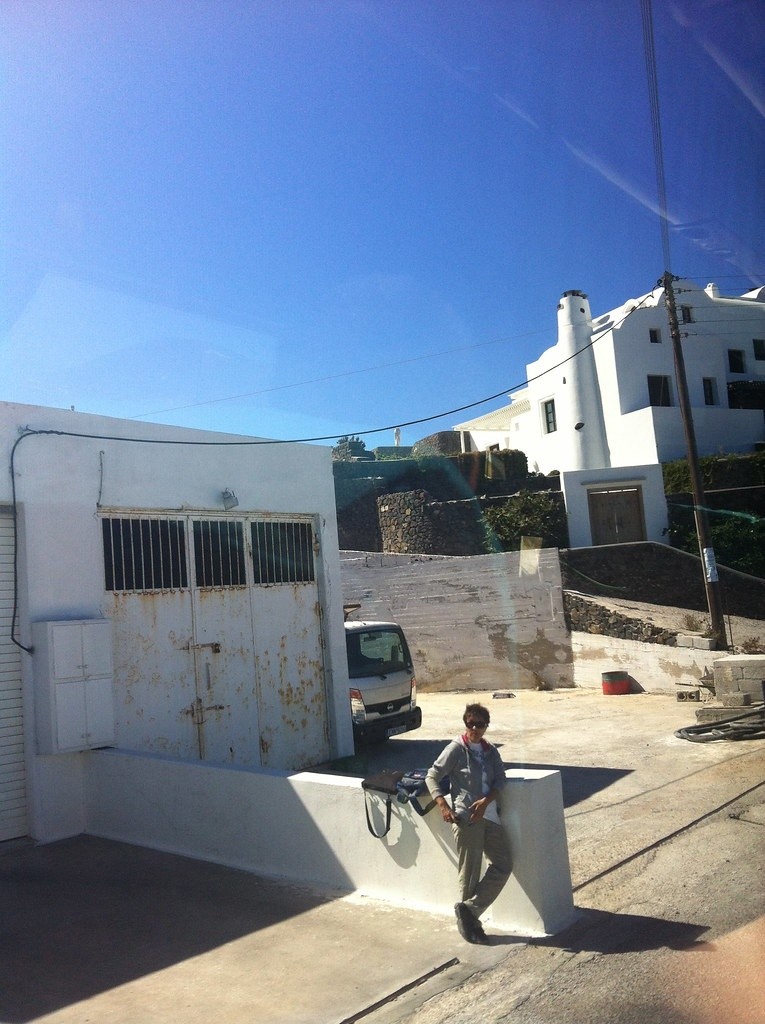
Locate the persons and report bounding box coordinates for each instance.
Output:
[424,704,508,944]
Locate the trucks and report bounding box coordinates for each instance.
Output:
[343,620,423,745]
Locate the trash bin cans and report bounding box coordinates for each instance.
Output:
[601,671,629,695]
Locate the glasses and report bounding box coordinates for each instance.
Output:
[465,721,489,729]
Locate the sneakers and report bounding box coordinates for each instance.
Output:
[454,903,474,942]
[471,921,489,945]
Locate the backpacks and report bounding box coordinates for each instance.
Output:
[397,769,451,816]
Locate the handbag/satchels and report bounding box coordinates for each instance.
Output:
[362,773,403,838]
